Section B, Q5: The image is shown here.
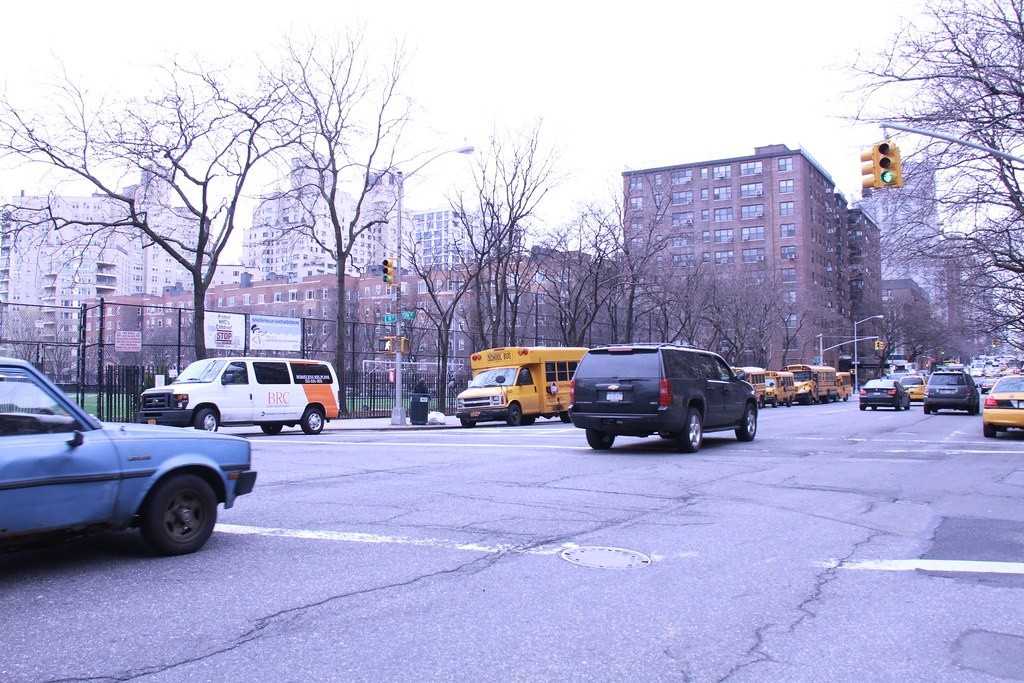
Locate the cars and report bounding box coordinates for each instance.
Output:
[983,376,1024,438]
[859,379,911,411]
[899,356,1024,402]
[0,357,258,556]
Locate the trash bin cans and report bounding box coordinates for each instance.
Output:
[408,393,432,426]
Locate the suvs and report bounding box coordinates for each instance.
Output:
[924,371,982,415]
[567,343,757,453]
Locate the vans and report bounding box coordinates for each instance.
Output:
[884,353,909,368]
[137,358,339,436]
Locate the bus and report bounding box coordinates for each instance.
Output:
[454,346,590,427]
[722,364,853,409]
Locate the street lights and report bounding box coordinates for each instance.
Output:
[855,315,883,394]
[362,145,473,424]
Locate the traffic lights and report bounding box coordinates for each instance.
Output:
[991,338,1001,347]
[381,258,391,285]
[401,335,410,355]
[377,336,394,354]
[860,143,904,191]
[875,341,884,351]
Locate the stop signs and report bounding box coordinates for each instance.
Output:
[550,383,558,397]
